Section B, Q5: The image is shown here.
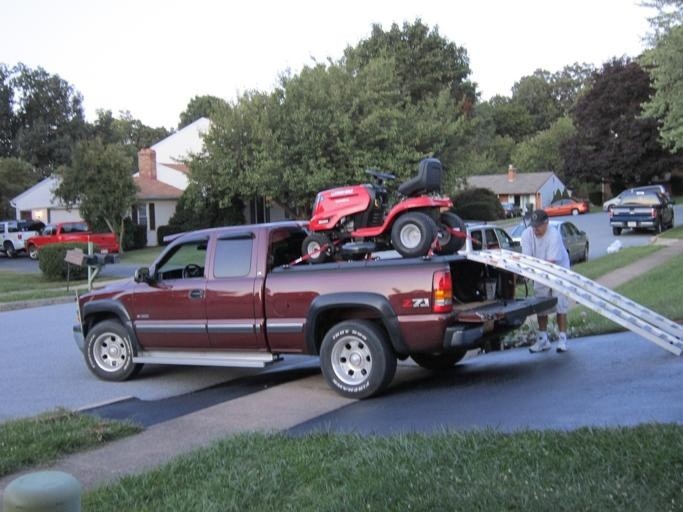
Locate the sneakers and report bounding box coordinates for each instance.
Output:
[529,337,551,352]
[556,334,568,353]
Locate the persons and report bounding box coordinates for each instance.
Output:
[519,208,571,355]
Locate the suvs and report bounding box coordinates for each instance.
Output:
[0,220,47,260]
[299,157,465,262]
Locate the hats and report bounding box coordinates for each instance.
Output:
[527,210,549,228]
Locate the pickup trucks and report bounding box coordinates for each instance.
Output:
[70,219,559,399]
[26,222,120,261]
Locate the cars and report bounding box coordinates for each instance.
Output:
[498,184,673,279]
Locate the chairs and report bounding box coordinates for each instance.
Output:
[396,157,442,197]
